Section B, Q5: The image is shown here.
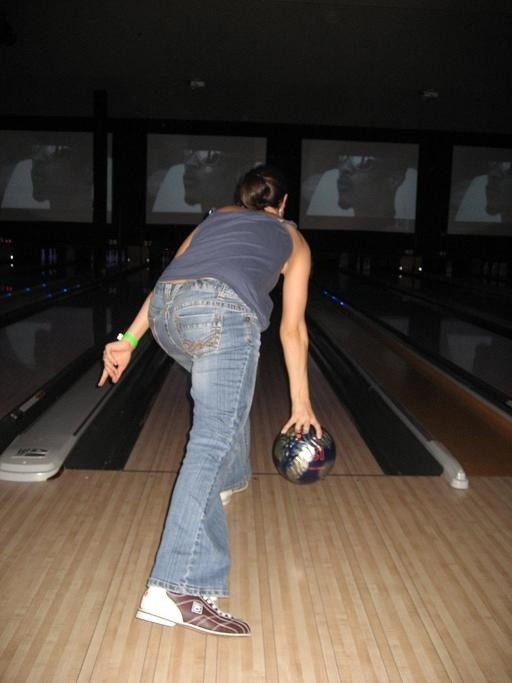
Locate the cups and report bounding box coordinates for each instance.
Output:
[29,141,77,160]
[328,148,382,169]
[489,156,512,176]
[176,144,225,165]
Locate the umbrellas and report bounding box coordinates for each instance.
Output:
[135,585,252,638]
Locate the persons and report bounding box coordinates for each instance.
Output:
[30,144,93,210]
[183,148,256,212]
[95,163,325,637]
[485,161,512,223]
[337,155,406,218]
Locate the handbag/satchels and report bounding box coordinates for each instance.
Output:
[121,333,139,348]
